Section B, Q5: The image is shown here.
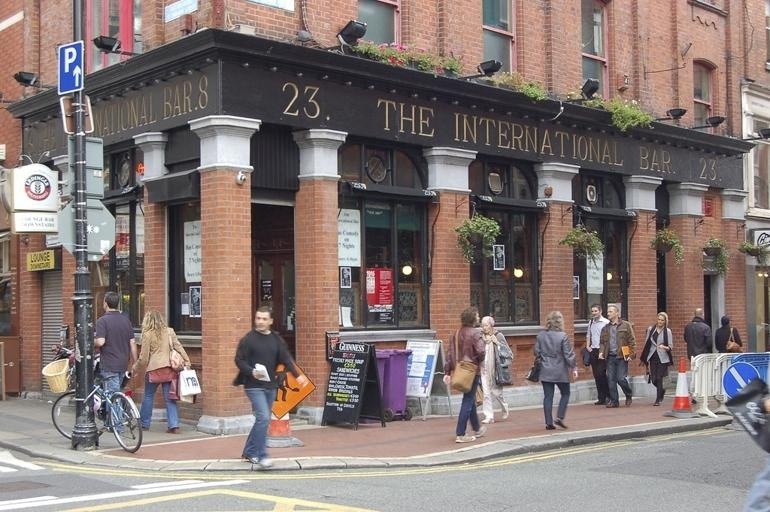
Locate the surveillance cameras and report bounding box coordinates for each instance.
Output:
[236,173,246,185]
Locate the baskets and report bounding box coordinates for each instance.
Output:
[42,358,70,393]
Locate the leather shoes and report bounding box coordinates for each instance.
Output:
[546,424,555,430]
[167,427,179,433]
[661,388,666,401]
[595,399,605,404]
[654,400,659,405]
[554,418,566,427]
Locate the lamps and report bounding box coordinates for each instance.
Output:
[743,128,770,141]
[14,71,50,90]
[335,21,368,55]
[691,116,726,130]
[576,79,599,104]
[94,35,139,58]
[463,60,504,81]
[656,109,687,120]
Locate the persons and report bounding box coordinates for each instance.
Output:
[132,310,191,434]
[479,316,513,424]
[94,292,137,434]
[715,316,742,352]
[640,312,673,406]
[684,308,712,361]
[232,305,304,464]
[533,310,578,429]
[599,304,636,408]
[443,308,486,443]
[585,304,610,405]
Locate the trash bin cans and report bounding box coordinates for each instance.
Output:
[365,349,412,422]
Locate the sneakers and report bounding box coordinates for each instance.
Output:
[242,452,259,463]
[475,426,487,437]
[481,418,495,423]
[626,394,632,405]
[502,402,509,419]
[606,401,619,407]
[456,432,476,443]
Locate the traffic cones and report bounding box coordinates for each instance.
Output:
[664,355,702,420]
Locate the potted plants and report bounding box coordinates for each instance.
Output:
[737,241,770,271]
[703,237,730,281]
[450,213,503,265]
[649,229,686,268]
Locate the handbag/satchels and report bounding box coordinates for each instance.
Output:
[525,341,541,382]
[168,327,184,371]
[169,366,201,403]
[495,363,512,386]
[450,361,478,393]
[726,327,743,353]
[644,365,650,384]
[583,350,591,366]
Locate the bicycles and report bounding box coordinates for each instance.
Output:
[42,341,142,454]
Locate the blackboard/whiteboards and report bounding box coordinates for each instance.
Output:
[322,348,370,425]
